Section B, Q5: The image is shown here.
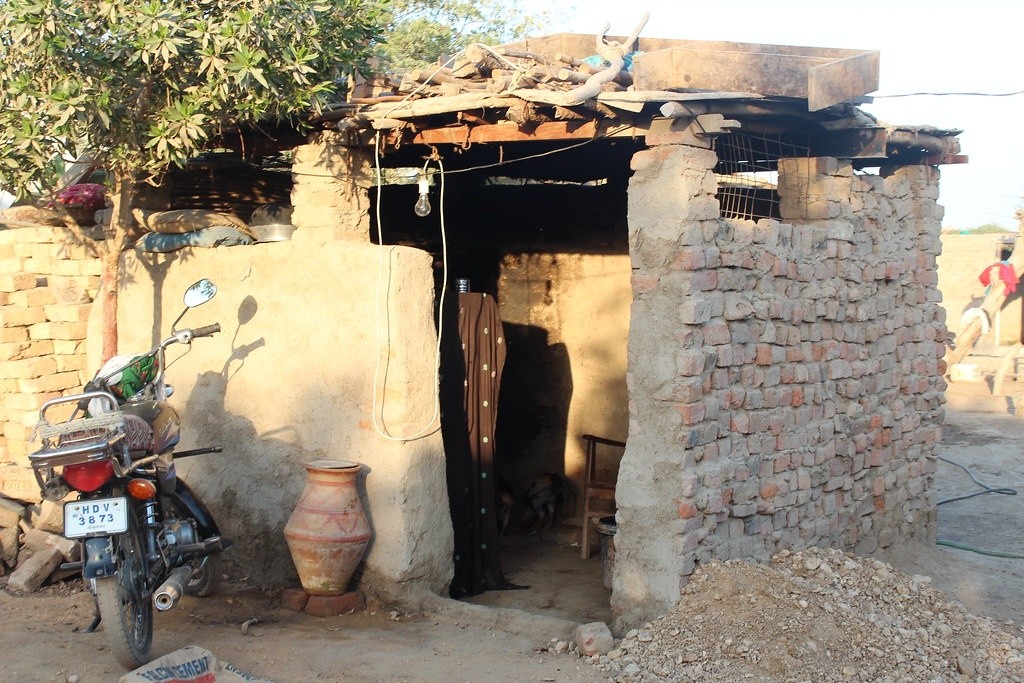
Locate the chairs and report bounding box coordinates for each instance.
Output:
[581,434,626,560]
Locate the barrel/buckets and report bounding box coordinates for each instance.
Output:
[594,515,618,590]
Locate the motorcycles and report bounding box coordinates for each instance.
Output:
[28,278,224,671]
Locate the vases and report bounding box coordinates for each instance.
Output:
[282,464,372,595]
